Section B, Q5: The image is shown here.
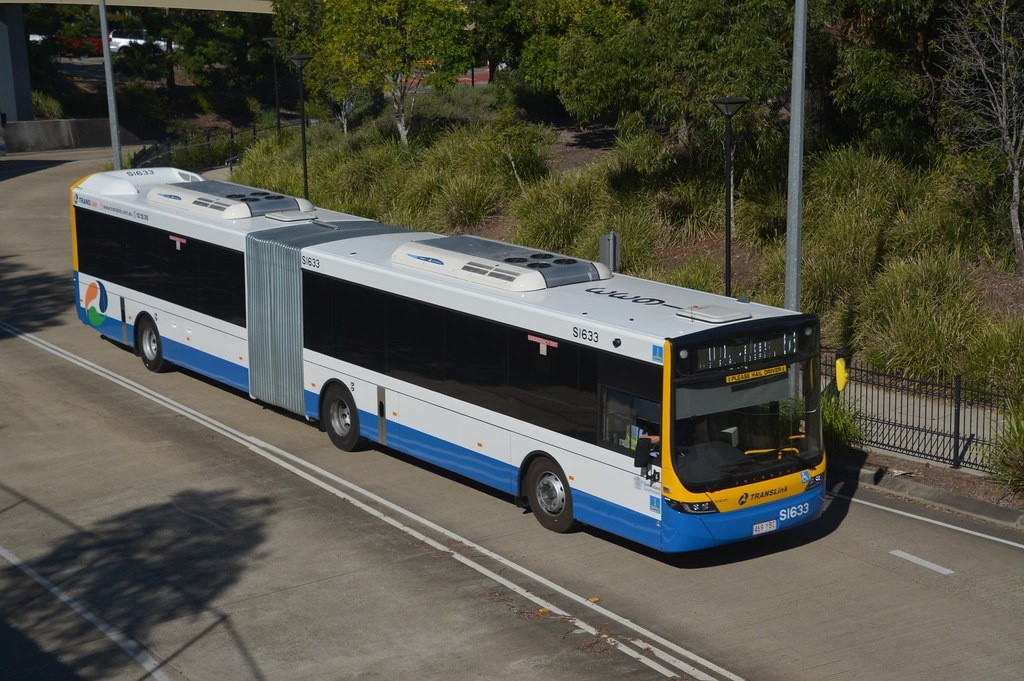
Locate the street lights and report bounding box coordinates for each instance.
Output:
[261,38,286,135]
[708,97,751,297]
[286,55,314,200]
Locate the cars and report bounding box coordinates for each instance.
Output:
[108,29,182,58]
[53,26,104,59]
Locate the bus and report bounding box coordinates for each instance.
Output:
[65,166,850,553]
